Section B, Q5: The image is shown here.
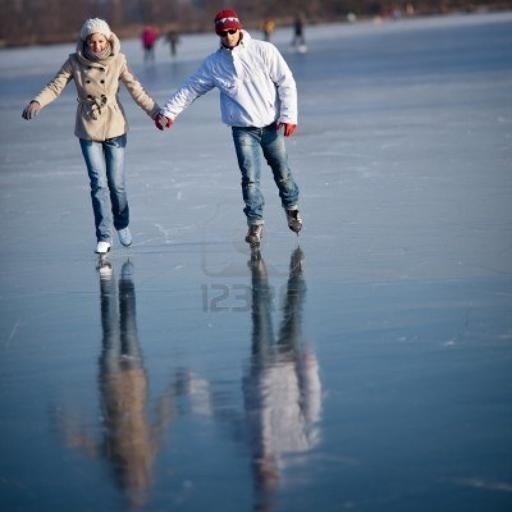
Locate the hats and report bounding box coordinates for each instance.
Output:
[80,18,111,42]
[214,9,240,34]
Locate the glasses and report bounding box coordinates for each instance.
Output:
[217,28,237,37]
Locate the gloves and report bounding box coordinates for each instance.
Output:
[22,101,41,120]
[155,113,173,130]
[277,123,296,136]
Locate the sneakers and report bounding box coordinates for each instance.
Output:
[290,248,305,274]
[249,260,265,272]
[117,227,132,247]
[96,264,113,281]
[94,241,112,254]
[245,224,264,243]
[122,263,135,279]
[286,209,302,232]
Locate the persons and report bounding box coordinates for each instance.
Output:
[346,9,359,24]
[261,13,276,43]
[184,248,326,510]
[163,24,180,59]
[139,20,160,64]
[372,0,512,24]
[288,10,308,54]
[79,261,182,512]
[20,16,167,254]
[154,5,304,242]
